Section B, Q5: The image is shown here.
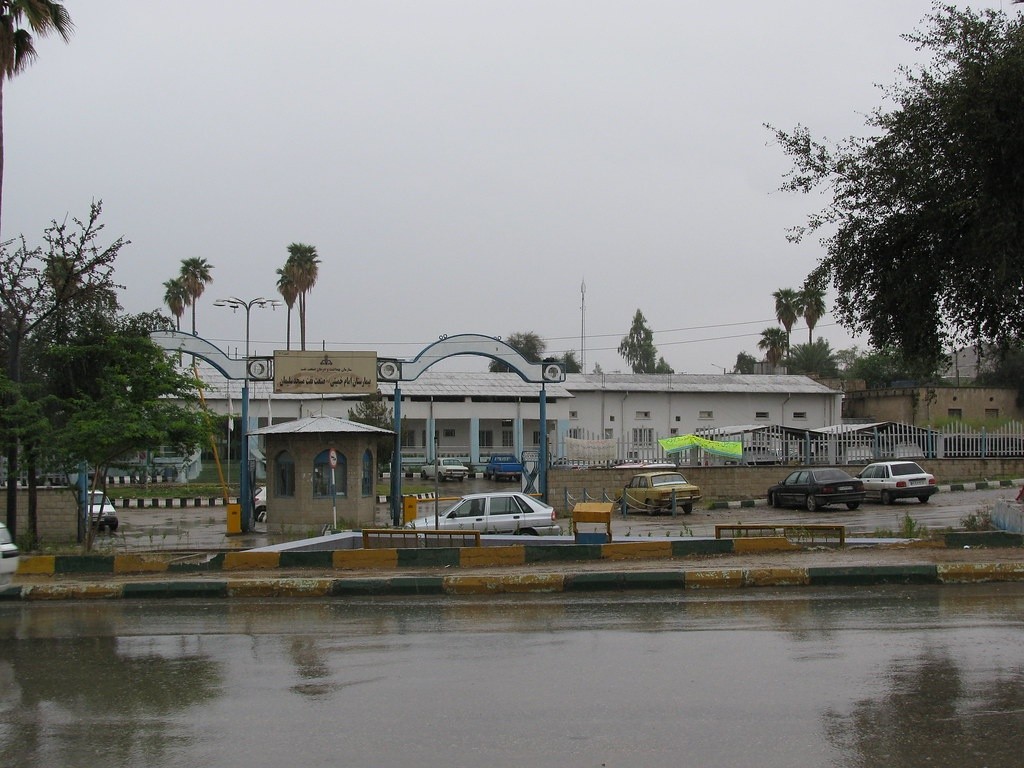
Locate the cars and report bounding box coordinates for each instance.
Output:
[420,457,469,482]
[254,486,266,523]
[87,491,118,531]
[403,491,564,537]
[616,471,703,516]
[853,462,935,505]
[485,452,524,482]
[768,467,866,512]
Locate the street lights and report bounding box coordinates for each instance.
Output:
[212,294,284,533]
